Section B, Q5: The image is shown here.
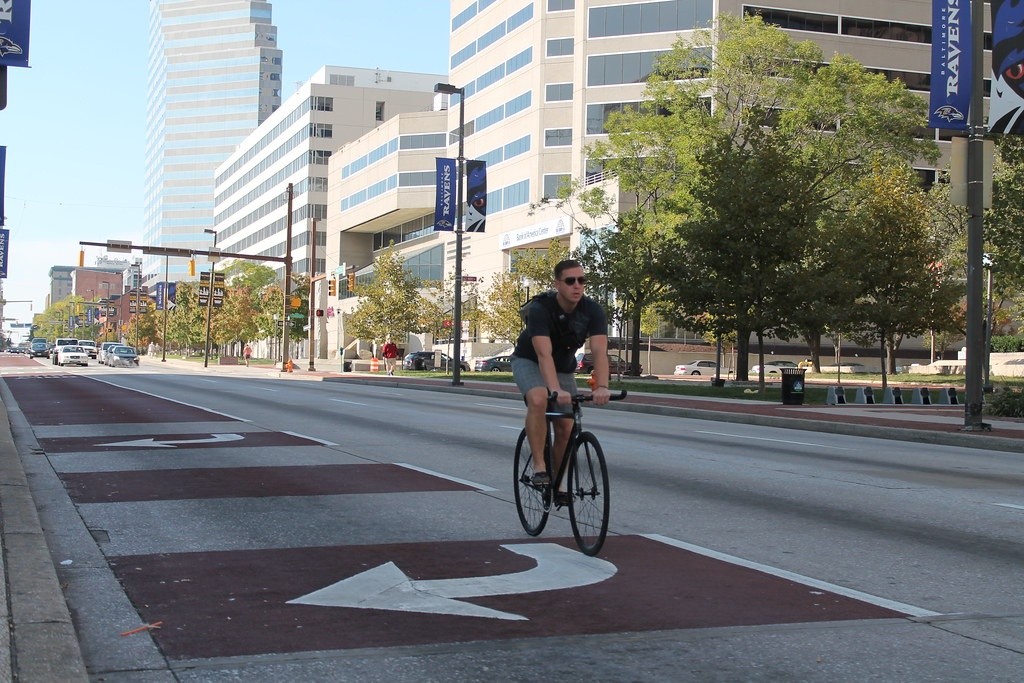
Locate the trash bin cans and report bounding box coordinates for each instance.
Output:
[344,360,352,372]
[779,367,807,405]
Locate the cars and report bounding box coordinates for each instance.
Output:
[96,342,140,368]
[56,345,89,366]
[7,347,30,354]
[475,356,512,372]
[752,361,798,373]
[673,360,736,375]
[28,343,51,359]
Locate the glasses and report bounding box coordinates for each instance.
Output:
[557,277,586,286]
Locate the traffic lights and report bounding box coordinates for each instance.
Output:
[346,274,355,291]
[328,279,336,296]
[316,310,323,317]
[188,260,195,276]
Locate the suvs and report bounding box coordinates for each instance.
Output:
[49,344,56,353]
[52,338,82,365]
[403,351,471,372]
[575,353,643,376]
[78,340,97,359]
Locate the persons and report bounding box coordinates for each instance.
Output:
[382,337,400,375]
[148,342,161,359]
[510,261,610,506]
[243,345,252,368]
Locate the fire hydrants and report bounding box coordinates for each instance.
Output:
[285,358,294,372]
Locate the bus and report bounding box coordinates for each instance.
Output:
[32,338,46,343]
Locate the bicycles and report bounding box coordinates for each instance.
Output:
[513,390,627,557]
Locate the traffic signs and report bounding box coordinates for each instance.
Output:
[278,320,296,327]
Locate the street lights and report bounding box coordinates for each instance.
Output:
[131,264,140,354]
[203,229,218,368]
[115,272,123,343]
[87,289,94,341]
[435,83,465,387]
[524,278,531,301]
[56,301,76,337]
[102,281,110,341]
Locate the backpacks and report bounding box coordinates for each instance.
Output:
[519,293,559,328]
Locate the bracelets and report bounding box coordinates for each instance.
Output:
[599,386,608,388]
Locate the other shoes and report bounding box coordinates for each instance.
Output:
[532,471,550,484]
[388,372,393,376]
[559,490,576,503]
